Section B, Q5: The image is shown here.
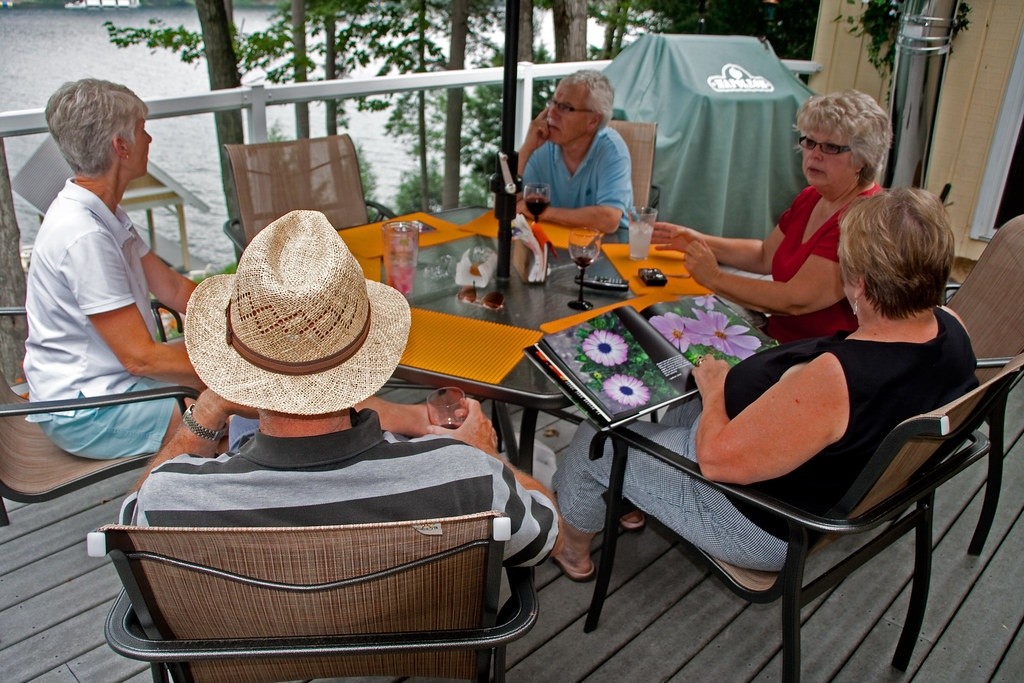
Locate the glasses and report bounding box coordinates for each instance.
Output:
[799,133,852,155]
[546,96,593,113]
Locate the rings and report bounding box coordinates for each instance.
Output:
[698,359,706,365]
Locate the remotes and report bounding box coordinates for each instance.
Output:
[574,275,629,292]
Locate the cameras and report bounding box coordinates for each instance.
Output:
[638,268,668,286]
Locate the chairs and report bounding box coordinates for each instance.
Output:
[1,298,198,502]
[225,135,395,255]
[944,212,1024,557]
[95,512,540,682]
[586,351,1024,683]
[609,119,659,219]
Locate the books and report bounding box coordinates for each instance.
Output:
[523,293,781,432]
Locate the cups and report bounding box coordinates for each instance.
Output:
[628,206,658,262]
[381,221,417,296]
[426,386,468,429]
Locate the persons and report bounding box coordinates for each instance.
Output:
[22,80,239,460]
[550,186,978,584]
[118,209,566,572]
[519,70,640,235]
[649,89,895,350]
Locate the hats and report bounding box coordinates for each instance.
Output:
[183,209,411,415]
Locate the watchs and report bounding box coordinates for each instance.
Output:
[182,403,229,441]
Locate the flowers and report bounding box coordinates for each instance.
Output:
[573,295,763,409]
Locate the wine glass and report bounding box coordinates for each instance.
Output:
[567,227,601,311]
[523,182,550,223]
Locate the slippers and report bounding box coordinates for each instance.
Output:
[617,516,644,534]
[553,533,595,581]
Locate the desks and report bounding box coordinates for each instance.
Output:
[337,207,770,479]
